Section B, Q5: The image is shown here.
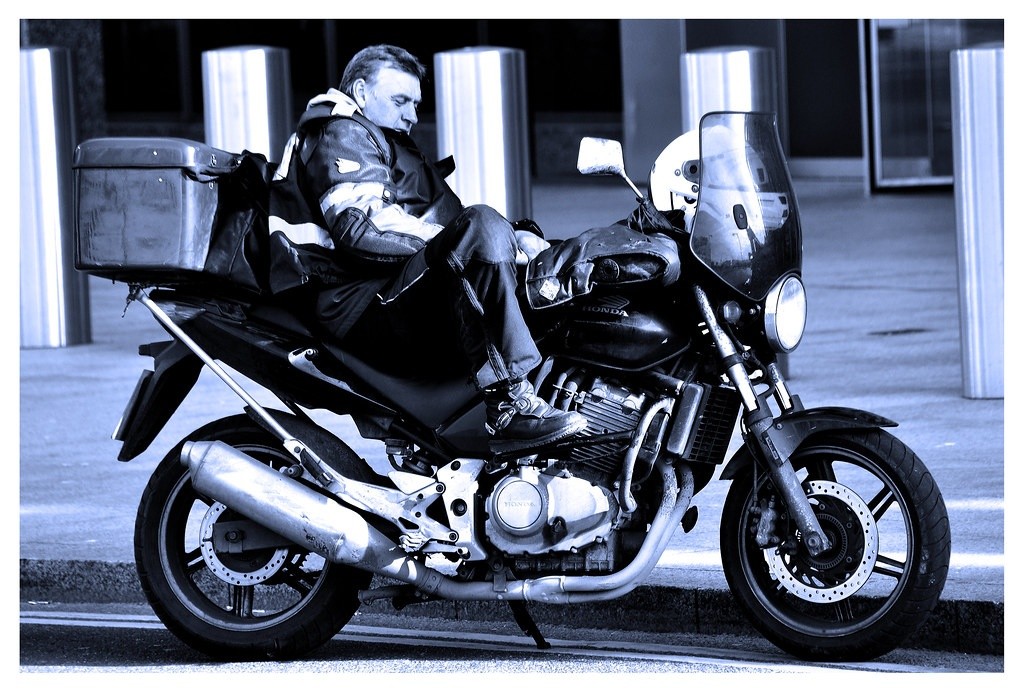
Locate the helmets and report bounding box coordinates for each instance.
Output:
[648,123,789,236]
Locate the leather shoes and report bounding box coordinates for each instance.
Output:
[484,374,588,452]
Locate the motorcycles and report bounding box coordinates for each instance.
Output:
[70,109,953,662]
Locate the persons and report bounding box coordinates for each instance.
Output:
[269,43,587,456]
